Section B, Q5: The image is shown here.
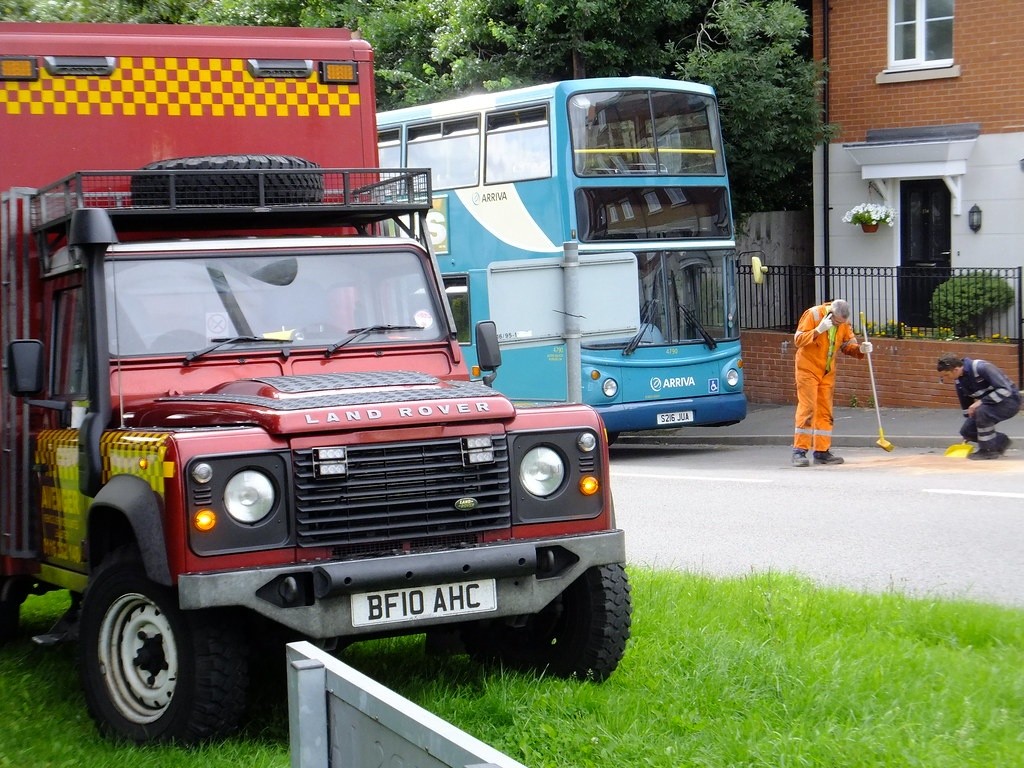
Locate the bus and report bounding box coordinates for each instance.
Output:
[369,76,769,447]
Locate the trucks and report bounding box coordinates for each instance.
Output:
[0,21,630,747]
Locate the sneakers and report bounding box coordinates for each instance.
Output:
[792,448,809,466]
[813,451,844,464]
[970,449,999,460]
[997,436,1013,455]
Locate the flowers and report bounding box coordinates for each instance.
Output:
[841,202,895,228]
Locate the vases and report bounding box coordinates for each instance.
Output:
[860,221,879,232]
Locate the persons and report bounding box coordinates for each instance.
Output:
[937,353,1022,461]
[792,300,873,467]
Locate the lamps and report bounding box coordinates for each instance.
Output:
[968,203,982,233]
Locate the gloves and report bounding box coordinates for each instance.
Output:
[815,313,833,334]
[859,342,872,354]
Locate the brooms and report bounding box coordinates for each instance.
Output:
[859,311,896,453]
